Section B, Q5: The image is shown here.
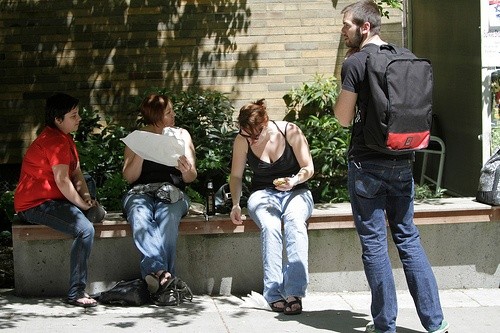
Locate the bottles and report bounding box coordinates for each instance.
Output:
[206,179,215,215]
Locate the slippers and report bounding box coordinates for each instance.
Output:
[269,299,289,312]
[283,296,302,315]
[63,293,97,307]
[83,206,106,223]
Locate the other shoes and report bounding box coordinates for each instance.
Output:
[366,324,377,333]
[144,271,186,296]
[428,322,448,333]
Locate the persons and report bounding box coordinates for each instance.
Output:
[230,98,315,315]
[334,0,449,333]
[13,93,106,307]
[122,93,197,306]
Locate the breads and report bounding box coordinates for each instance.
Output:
[273,178,286,186]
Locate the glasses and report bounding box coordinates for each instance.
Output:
[239,126,263,141]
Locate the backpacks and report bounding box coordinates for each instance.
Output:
[357,43,432,154]
[476,150,499,206]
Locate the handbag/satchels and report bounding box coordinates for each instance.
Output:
[99,279,149,307]
